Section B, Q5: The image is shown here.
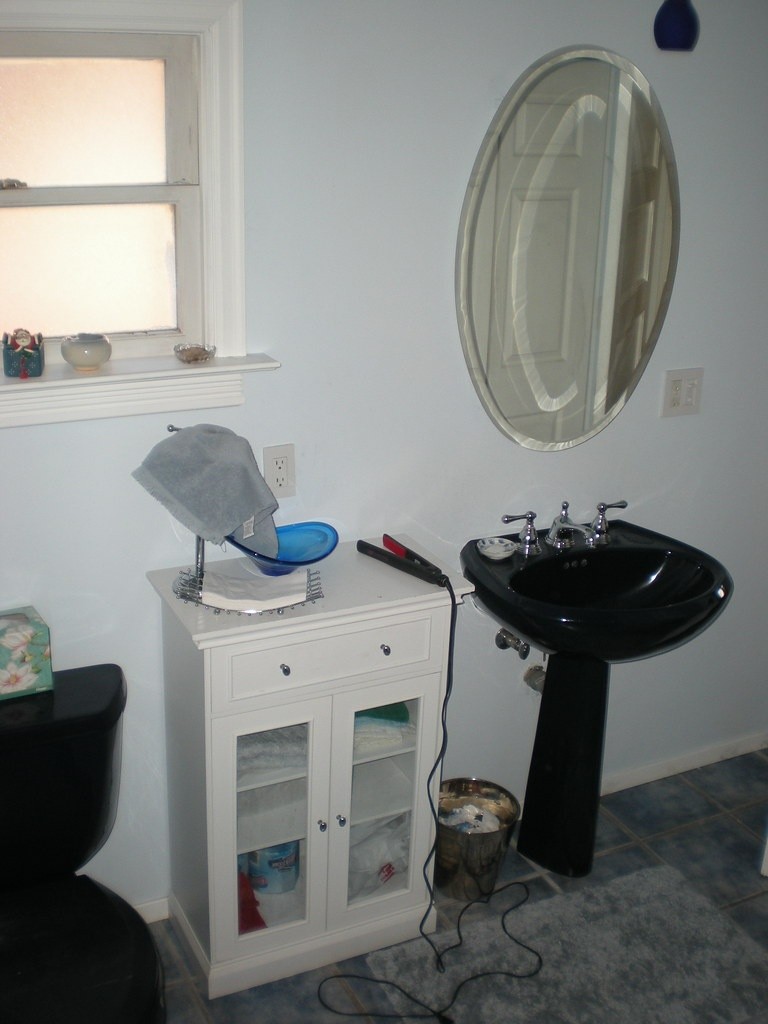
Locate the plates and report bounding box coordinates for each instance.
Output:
[477,538,517,560]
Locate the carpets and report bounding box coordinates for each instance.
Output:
[362,865,768,1024]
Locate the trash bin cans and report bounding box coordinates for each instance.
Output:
[433,775,522,903]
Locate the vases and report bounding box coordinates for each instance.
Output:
[61,333,112,371]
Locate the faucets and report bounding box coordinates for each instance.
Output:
[543,501,600,551]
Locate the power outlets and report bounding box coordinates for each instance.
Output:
[263,443,296,498]
[662,368,703,418]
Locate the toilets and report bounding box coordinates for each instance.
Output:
[1,664,174,1024]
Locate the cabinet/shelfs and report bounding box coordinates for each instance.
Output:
[147,532,476,1001]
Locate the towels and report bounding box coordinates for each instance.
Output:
[131,422,282,559]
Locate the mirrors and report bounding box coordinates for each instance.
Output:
[455,44,681,451]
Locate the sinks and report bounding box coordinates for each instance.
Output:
[460,518,737,666]
